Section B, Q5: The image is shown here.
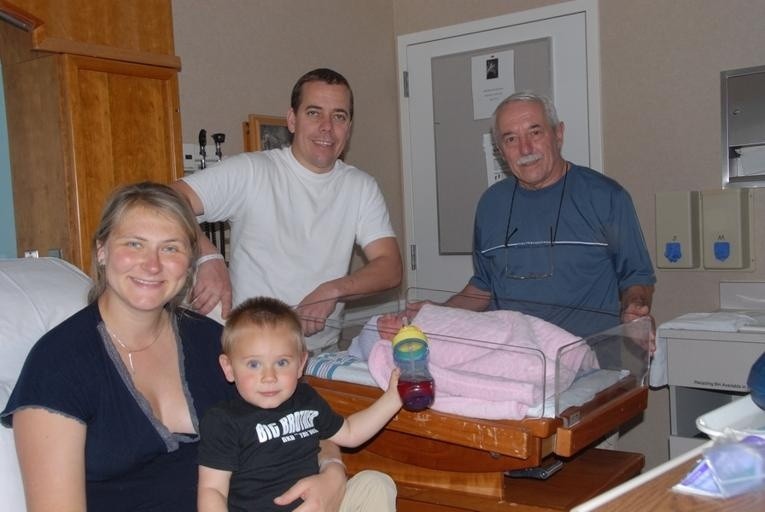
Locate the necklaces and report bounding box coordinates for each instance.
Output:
[104,319,168,375]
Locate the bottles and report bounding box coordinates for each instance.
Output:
[392,315,436,414]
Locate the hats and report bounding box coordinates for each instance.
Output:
[348,315,383,359]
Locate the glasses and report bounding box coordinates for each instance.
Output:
[505,227,555,279]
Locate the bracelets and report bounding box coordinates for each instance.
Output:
[317,457,348,474]
[195,254,226,267]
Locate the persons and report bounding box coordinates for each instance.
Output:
[0,180,401,512]
[194,294,403,512]
[346,309,409,357]
[164,68,406,357]
[399,90,659,373]
